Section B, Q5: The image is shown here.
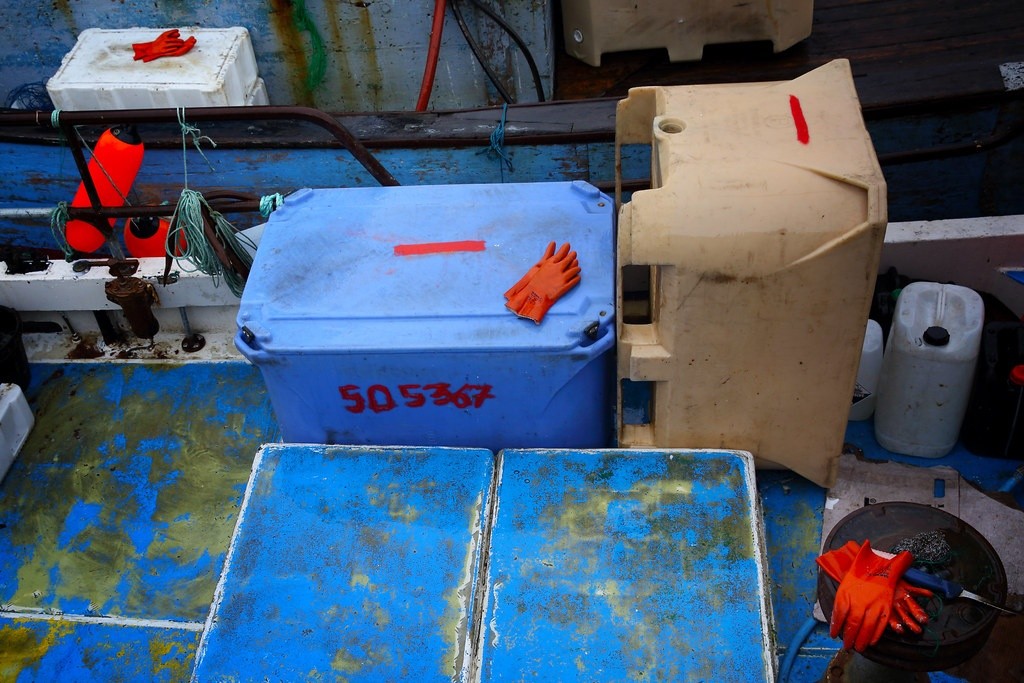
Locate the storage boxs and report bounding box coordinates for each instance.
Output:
[245,77,271,107]
[232,178,617,453]
[614,57,890,492]
[562,0,816,68]
[45,27,260,111]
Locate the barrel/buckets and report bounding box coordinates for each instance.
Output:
[874,282,985,459]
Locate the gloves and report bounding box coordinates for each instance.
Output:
[132,29,197,64]
[504,240,581,325]
[814,539,934,652]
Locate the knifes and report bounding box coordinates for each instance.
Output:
[902,569,1017,614]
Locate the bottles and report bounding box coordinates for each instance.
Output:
[847,318,885,419]
